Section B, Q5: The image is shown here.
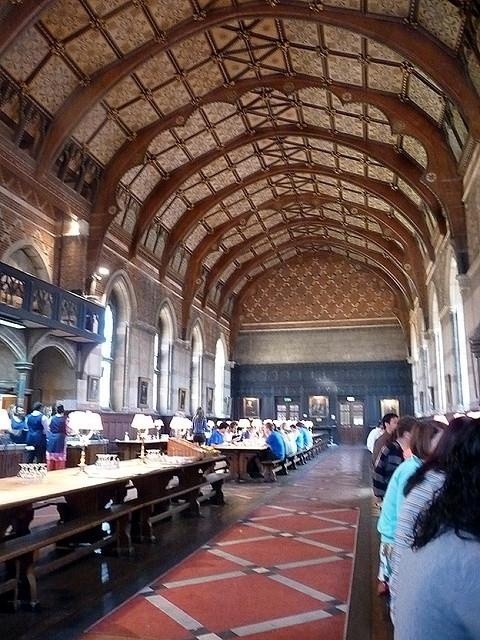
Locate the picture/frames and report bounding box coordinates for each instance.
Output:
[309,395,330,419]
[379,399,400,420]
[86,375,101,402]
[206,387,213,413]
[137,377,152,409]
[242,397,261,419]
[178,387,188,411]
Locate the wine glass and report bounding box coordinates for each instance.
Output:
[144,449,167,464]
[16,463,47,484]
[94,452,119,470]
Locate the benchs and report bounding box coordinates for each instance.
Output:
[0,473,230,611]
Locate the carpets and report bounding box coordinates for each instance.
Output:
[72,443,365,640]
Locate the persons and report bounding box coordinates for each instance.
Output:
[7,401,80,470]
[365,413,480,639]
[207,421,313,479]
[191,408,207,446]
